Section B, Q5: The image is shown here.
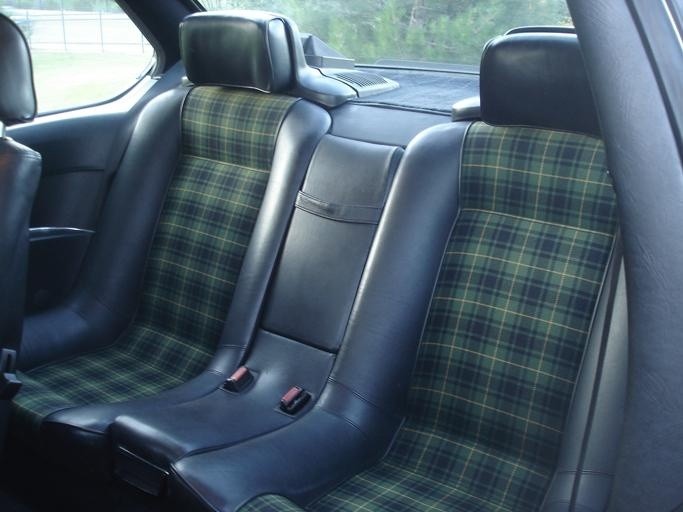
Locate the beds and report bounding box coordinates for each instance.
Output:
[155,24,631,511]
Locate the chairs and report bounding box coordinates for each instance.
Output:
[1,7,332,511]
